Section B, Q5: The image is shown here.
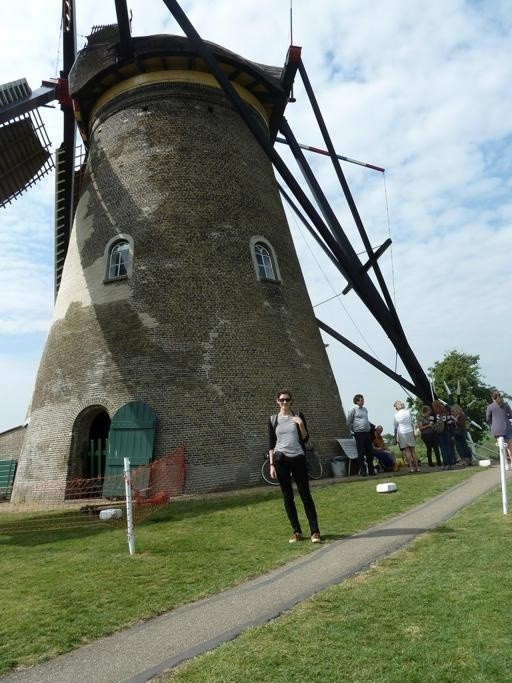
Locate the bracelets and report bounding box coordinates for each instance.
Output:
[270,464,274,466]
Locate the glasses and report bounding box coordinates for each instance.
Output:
[277,399,291,402]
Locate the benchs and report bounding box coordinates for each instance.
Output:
[336,438,379,476]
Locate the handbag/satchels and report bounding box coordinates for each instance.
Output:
[275,451,284,461]
[464,418,471,431]
[432,420,444,433]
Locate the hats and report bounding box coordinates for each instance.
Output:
[421,405,432,416]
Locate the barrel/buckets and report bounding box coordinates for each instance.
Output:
[329,455,346,476]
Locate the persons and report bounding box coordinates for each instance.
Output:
[417,400,476,470]
[371,428,401,472]
[394,400,421,474]
[347,394,376,476]
[375,425,396,472]
[486,391,512,471]
[267,390,321,544]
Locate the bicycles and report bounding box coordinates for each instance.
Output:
[261,446,323,485]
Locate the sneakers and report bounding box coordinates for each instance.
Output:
[408,462,456,473]
[288,532,301,543]
[311,533,320,543]
